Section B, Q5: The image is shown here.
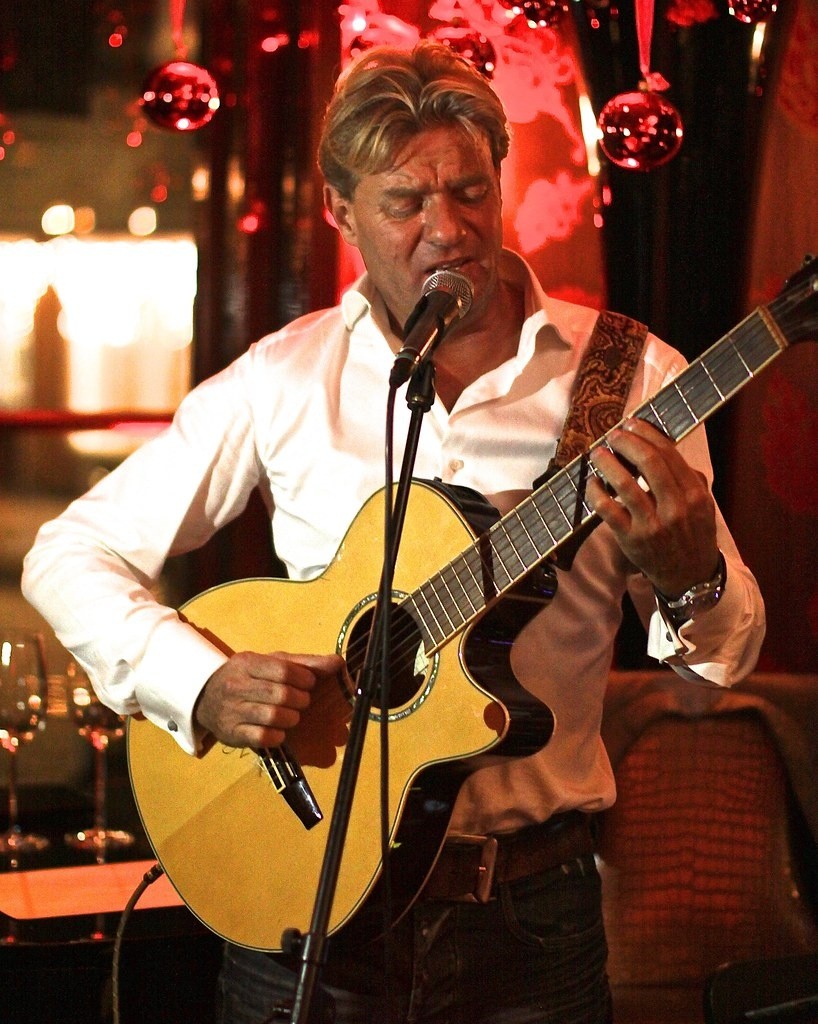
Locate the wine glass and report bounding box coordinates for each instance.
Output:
[64,656,136,850]
[0,632,51,854]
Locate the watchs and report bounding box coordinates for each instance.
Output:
[667,552,727,618]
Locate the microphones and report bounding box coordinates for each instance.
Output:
[388,270,475,391]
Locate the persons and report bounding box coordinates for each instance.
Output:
[15,37,765,1024]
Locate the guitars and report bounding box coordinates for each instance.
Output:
[123,251,818,959]
